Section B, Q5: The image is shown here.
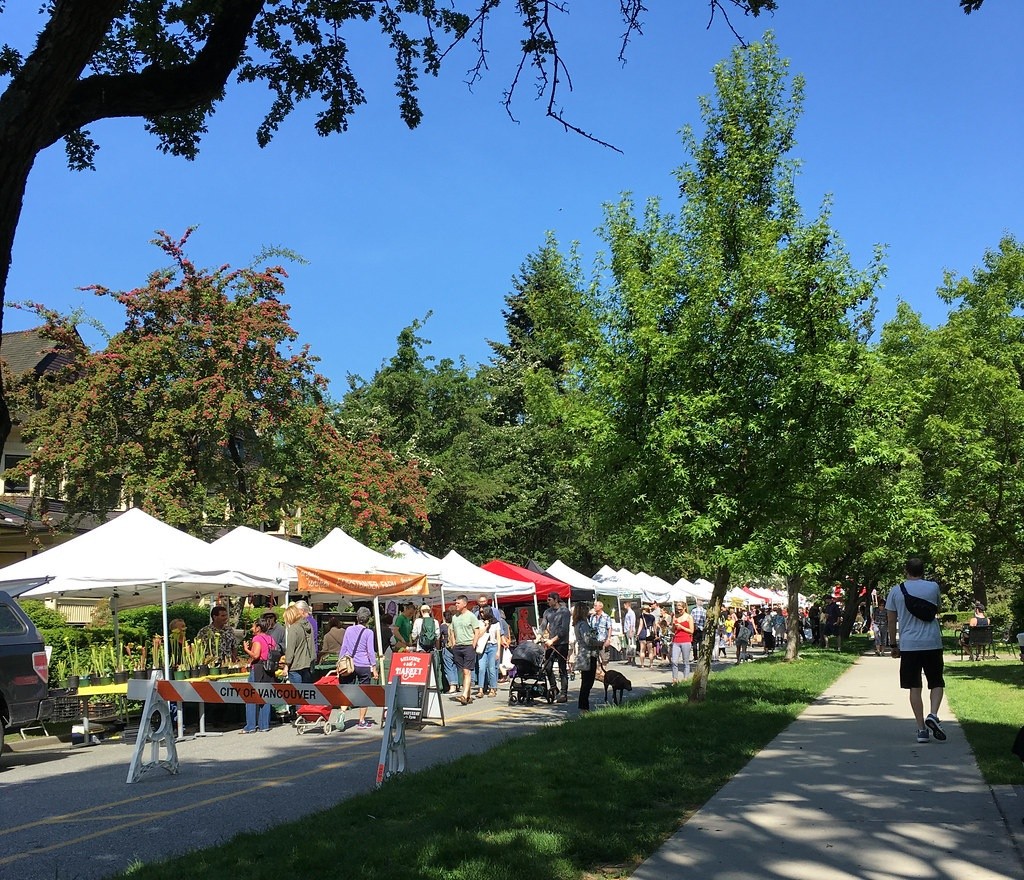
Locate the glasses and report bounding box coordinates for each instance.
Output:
[546,598,554,602]
[878,604,885,606]
[524,612,529,614]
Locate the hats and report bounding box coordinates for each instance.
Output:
[972,603,985,611]
[821,594,832,601]
[548,593,564,603]
[420,604,431,611]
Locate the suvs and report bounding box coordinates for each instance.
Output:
[0,576,56,753]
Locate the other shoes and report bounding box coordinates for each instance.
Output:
[976,656,981,661]
[881,652,886,656]
[618,652,778,671]
[967,657,974,662]
[173,711,375,743]
[445,671,592,714]
[875,650,880,656]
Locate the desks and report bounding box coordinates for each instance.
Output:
[66,669,284,748]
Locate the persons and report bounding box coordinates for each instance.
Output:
[167,593,889,734]
[884,558,946,742]
[959,604,990,661]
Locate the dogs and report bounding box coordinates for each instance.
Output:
[736,651,753,663]
[600,662,632,705]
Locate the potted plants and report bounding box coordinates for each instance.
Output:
[56,629,254,688]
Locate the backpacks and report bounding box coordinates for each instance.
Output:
[257,635,281,672]
[418,617,438,651]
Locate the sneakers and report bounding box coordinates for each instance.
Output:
[925,713,947,741]
[917,728,930,742]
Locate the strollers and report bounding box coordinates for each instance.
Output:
[508,641,556,706]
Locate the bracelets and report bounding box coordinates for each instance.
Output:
[890,644,897,648]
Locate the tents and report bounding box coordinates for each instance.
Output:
[0,506,807,742]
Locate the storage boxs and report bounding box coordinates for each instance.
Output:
[46,695,80,722]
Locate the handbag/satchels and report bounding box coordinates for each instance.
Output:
[866,627,875,641]
[904,593,938,623]
[476,620,490,654]
[626,637,635,658]
[336,655,354,677]
[502,646,514,663]
[575,642,590,671]
[646,614,790,646]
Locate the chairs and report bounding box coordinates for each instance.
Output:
[936,614,958,636]
[959,624,997,661]
[1000,619,1024,659]
